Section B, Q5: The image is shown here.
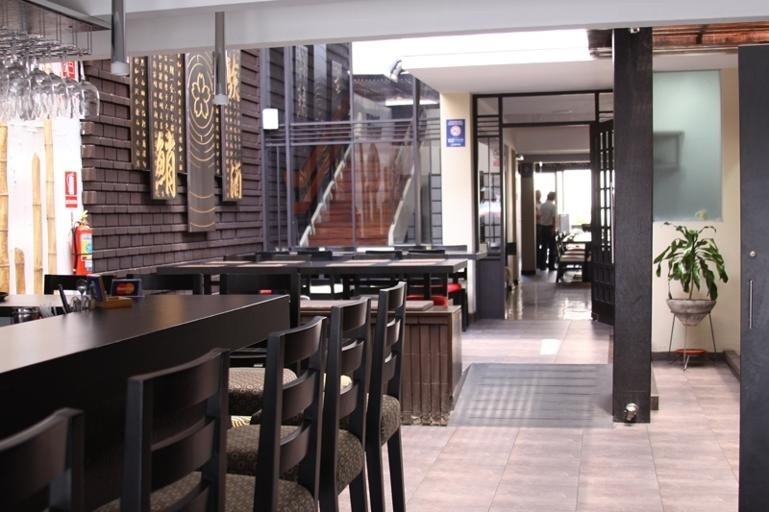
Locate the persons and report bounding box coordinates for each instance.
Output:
[539,191,559,272]
[534,190,544,252]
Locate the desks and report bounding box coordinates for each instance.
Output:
[0,295,291,422]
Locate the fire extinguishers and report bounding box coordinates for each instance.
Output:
[73,210,93,275]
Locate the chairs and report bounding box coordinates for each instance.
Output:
[553,230,592,285]
[0,242,467,330]
[250,280,406,511]
[98,316,329,509]
[218,271,300,415]
[226,296,372,511]
[1,407,86,512]
[120,348,228,509]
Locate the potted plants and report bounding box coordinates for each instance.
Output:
[653,218,729,329]
